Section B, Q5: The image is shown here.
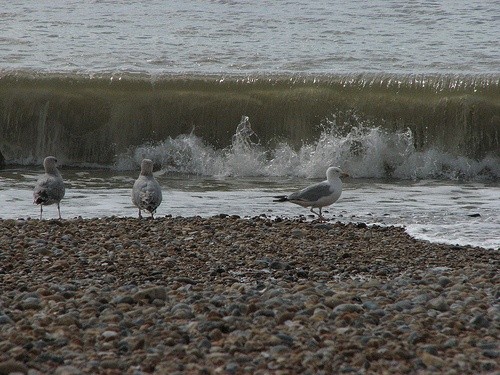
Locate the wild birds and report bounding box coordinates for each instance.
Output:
[132,158,163,218]
[273,166,349,220]
[32,156,66,219]
